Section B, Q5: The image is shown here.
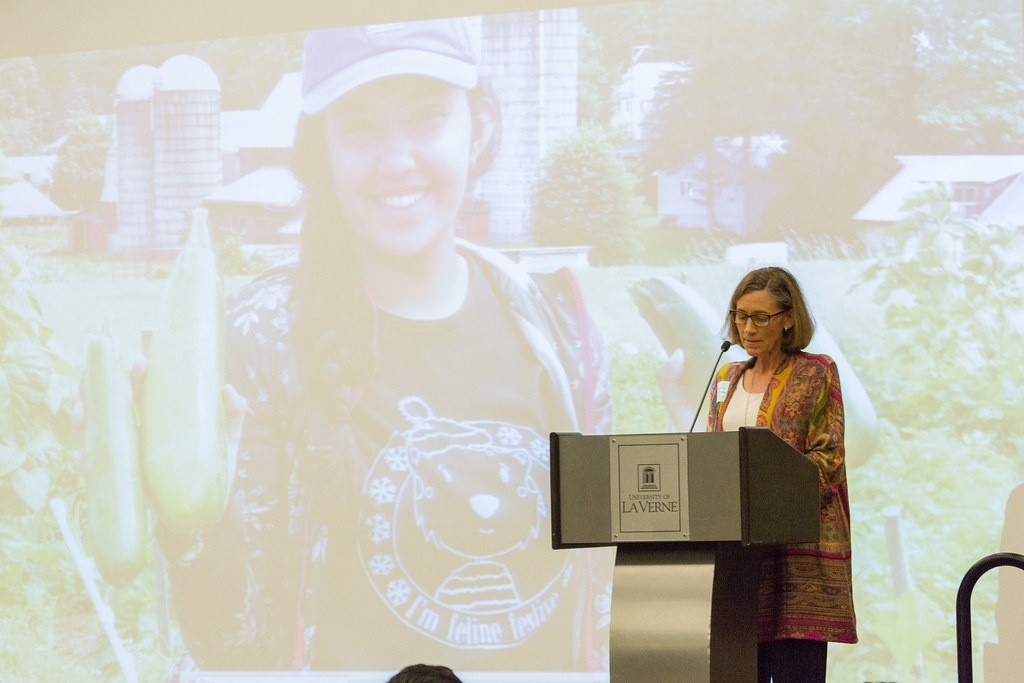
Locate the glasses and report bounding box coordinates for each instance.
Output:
[730,308,791,328]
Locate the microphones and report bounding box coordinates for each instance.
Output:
[691,341,732,433]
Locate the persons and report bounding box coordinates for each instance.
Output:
[141,21,617,668]
[387,664,462,683]
[707,267,859,683]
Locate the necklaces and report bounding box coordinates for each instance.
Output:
[744,369,755,425]
